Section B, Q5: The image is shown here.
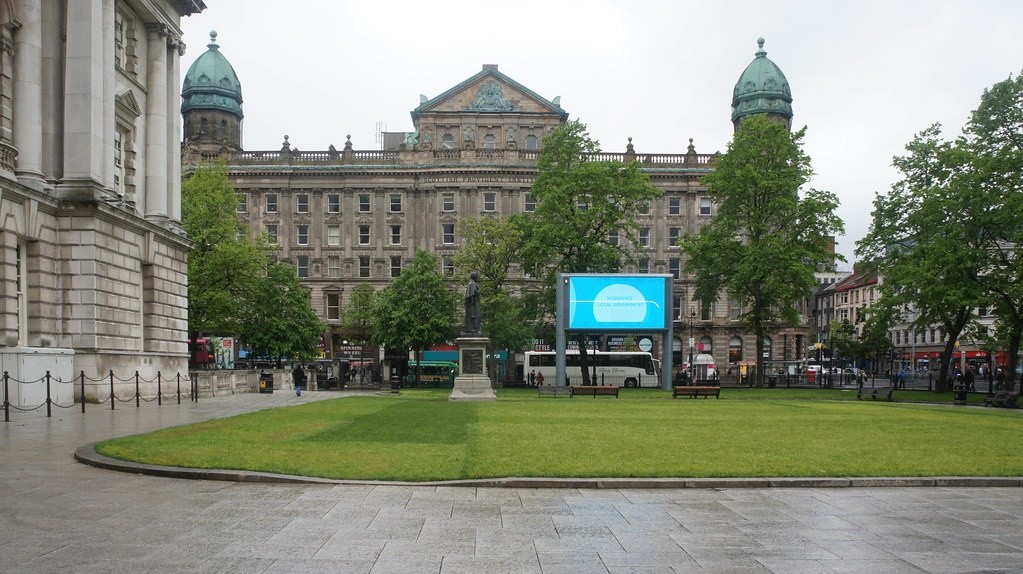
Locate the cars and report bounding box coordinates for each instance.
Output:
[808,364,867,382]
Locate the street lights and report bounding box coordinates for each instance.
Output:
[689,305,696,386]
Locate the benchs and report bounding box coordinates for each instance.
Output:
[572,385,619,399]
[672,385,720,400]
[696,380,720,386]
[502,381,527,388]
[538,386,573,399]
[983,391,1020,408]
[856,387,894,402]
[673,380,687,386]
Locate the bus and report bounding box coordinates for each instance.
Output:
[239,357,350,388]
[524,349,661,389]
[402,361,459,388]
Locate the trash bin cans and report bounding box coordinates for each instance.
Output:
[740,373,748,386]
[768,374,777,388]
[258,370,274,394]
[952,384,967,405]
[389,375,400,393]
[433,377,440,388]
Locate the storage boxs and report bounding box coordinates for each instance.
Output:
[259,373,274,394]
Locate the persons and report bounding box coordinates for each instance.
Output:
[713,367,720,380]
[530,370,536,386]
[892,369,906,389]
[676,370,687,381]
[727,368,733,383]
[536,371,544,387]
[857,370,867,392]
[464,272,483,334]
[292,364,306,396]
[350,366,357,382]
[953,365,1005,392]
